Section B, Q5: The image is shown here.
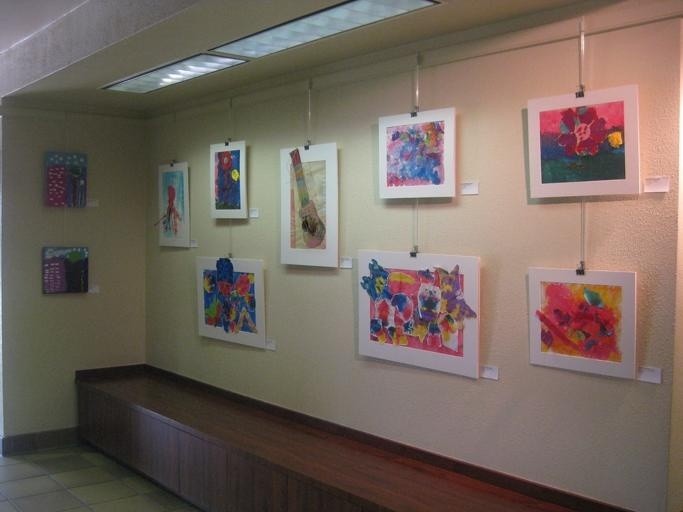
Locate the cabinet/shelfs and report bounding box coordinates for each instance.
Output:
[73,363,635,511]
